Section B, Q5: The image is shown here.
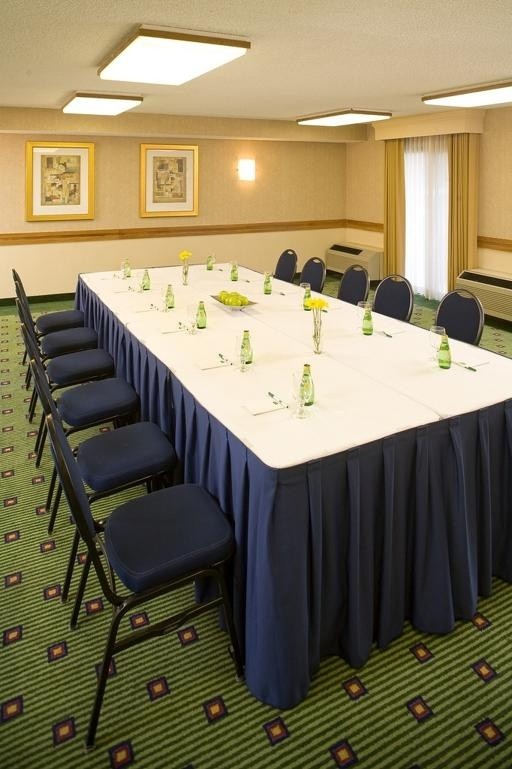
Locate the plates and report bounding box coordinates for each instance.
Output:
[211,294,257,310]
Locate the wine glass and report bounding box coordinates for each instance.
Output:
[356,300,371,334]
[234,336,250,373]
[428,325,445,364]
[299,283,311,312]
[290,371,312,419]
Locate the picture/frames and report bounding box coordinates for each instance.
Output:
[24,140,95,223]
[139,142,200,219]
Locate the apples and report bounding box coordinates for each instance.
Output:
[218,289,248,307]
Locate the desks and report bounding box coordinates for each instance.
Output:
[73,262,509,710]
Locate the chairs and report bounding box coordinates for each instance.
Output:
[334,262,366,305]
[430,283,489,351]
[271,248,297,285]
[18,326,148,511]
[12,268,100,389]
[24,361,183,606]
[15,301,131,450]
[42,416,258,745]
[368,271,418,323]
[296,256,328,291]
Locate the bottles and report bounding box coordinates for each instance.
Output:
[142,269,151,290]
[303,288,312,312]
[438,334,451,370]
[241,329,252,364]
[196,300,207,329]
[165,285,175,309]
[230,263,238,282]
[263,272,272,295]
[361,309,374,335]
[301,364,314,407]
[124,259,132,277]
[207,254,214,270]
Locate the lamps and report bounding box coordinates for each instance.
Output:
[95,24,252,87]
[58,90,143,117]
[295,107,393,128]
[422,78,512,110]
[236,159,256,182]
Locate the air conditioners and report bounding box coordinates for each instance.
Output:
[323,241,385,281]
[455,267,512,325]
